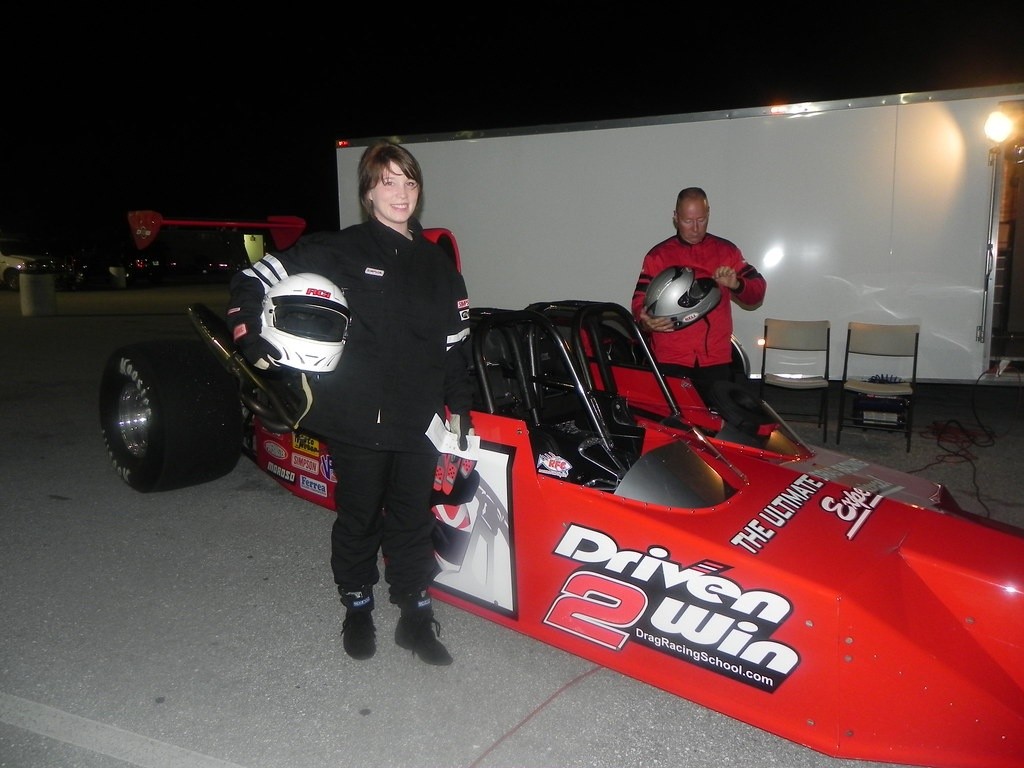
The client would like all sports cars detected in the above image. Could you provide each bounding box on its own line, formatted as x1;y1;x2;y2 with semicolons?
95;291;1024;768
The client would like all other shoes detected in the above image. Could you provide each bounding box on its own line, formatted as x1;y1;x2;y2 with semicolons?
395;606;453;666
341;610;377;660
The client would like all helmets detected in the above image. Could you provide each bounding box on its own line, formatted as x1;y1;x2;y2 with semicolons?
644;266;721;330
259;273;350;377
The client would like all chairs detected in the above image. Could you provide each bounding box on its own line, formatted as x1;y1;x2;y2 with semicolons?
836;321;922;455
759;317;831;444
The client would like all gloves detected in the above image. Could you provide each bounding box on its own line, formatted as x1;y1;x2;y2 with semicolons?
449;411;475;463
226;307;284;372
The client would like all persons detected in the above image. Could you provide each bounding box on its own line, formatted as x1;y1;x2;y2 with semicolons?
225;142;474;666
631;187;768;395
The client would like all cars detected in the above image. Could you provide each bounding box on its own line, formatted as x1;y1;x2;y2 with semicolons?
67;241;170;289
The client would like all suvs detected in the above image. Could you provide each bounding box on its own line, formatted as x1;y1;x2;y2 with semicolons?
1;239;76;294
